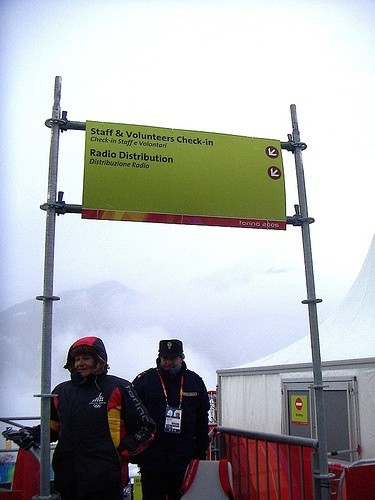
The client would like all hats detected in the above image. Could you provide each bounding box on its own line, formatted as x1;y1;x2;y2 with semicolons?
159;340;183;359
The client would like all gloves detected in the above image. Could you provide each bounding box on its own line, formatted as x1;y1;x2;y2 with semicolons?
6;427;40;451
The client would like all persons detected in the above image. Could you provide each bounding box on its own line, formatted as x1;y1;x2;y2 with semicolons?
12;336;150;500
130;338;212;500
